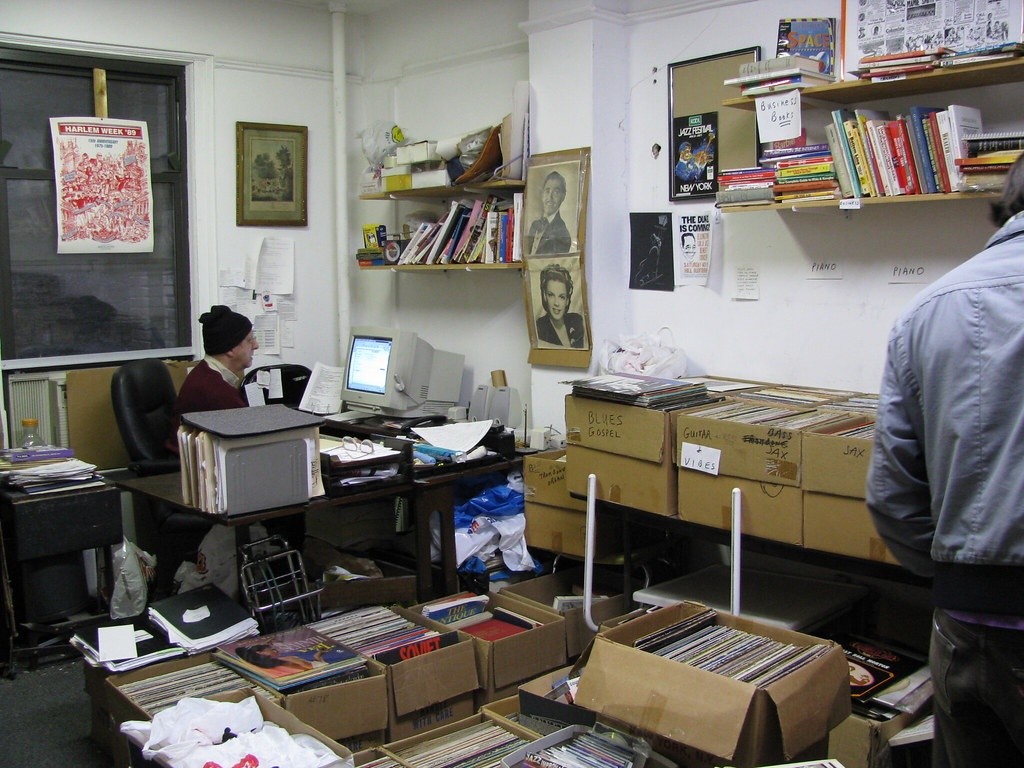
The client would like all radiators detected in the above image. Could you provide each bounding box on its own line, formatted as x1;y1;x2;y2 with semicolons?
6;369;61;449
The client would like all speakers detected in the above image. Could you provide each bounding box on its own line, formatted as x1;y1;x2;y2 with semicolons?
468;384;497;423
489;387;522;428
384;239;411;264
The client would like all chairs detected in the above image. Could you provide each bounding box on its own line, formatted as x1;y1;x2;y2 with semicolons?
551;531;679;608
110;358;215;597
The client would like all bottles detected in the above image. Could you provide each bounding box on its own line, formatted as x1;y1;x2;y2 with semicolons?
15;418;47;448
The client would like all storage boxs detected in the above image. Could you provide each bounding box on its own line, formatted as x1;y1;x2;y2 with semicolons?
319;556;417;611
361;222;387;249
412;169;452;190
381;165;412;192
395;139;441;164
82;374;922;768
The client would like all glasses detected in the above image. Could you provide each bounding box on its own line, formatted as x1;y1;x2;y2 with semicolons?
323;436;373;459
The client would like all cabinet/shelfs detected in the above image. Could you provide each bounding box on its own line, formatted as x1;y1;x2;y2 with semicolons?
358;178;524;273
721;57;1024;213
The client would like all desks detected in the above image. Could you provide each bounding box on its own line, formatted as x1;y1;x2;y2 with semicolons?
115;450;525;605
291;406;456;437
568;472;935;634
0;475;123;672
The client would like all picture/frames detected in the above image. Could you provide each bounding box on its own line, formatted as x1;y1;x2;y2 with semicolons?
667;46;764;202
235;121;308;227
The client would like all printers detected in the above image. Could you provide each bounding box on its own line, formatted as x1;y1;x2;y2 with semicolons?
320;433;413;499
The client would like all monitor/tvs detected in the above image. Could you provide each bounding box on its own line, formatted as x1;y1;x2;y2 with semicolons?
340;326;465;418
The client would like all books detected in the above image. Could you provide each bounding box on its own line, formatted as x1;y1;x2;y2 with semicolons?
557;372;880;439
356;193;524;266
858;42;1024;80
116;569;934;768
723;17;837;97
715;104;1024;209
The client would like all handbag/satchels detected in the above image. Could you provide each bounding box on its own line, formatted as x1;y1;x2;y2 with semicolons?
96;535;147;619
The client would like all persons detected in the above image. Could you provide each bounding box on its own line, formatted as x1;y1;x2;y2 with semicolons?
865;150;1024;768
169;304;326;583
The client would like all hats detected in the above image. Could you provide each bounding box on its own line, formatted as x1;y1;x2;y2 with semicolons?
198;305;252;355
